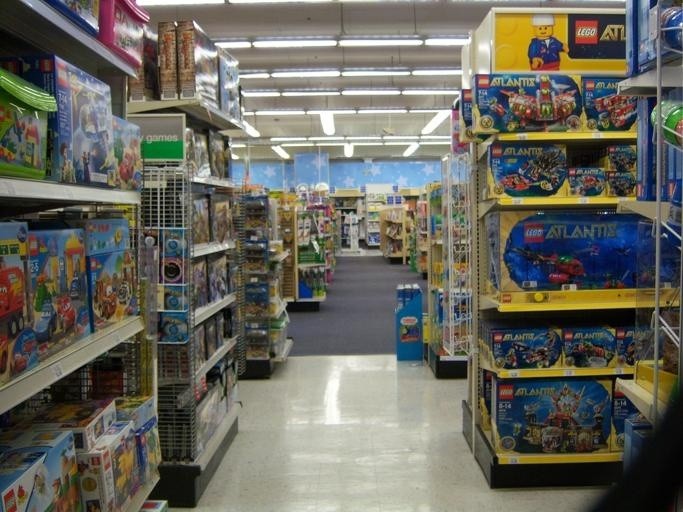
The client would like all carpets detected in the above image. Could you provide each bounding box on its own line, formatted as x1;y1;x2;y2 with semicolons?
280;256;428;356
